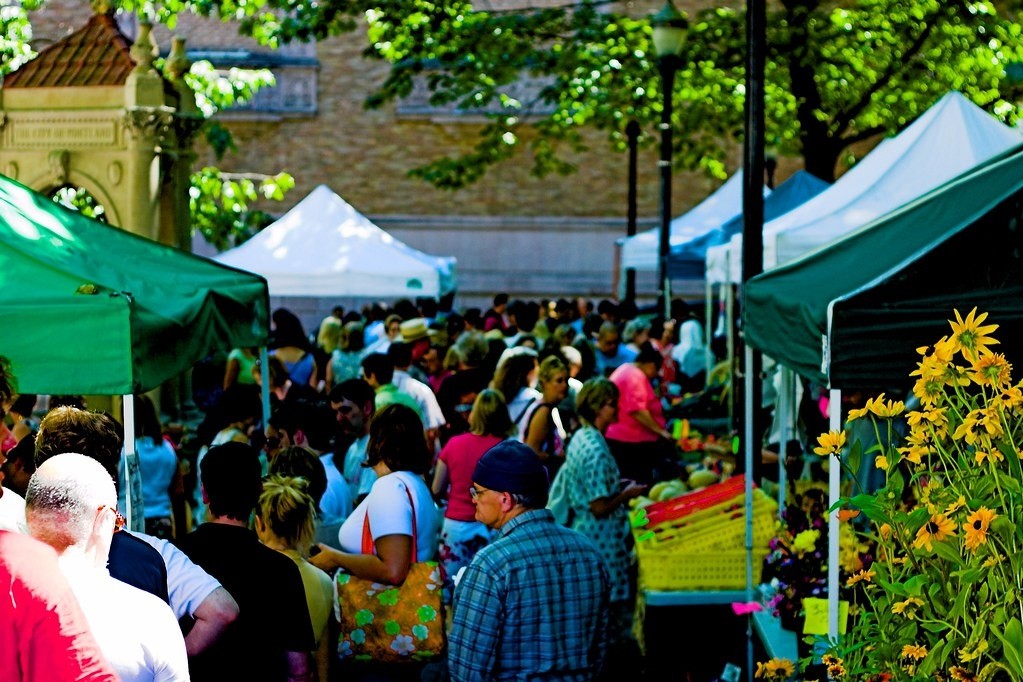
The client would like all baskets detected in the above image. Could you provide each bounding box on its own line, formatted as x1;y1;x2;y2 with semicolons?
628;475;778;590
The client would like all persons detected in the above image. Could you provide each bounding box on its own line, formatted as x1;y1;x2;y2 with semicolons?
0;294;905;682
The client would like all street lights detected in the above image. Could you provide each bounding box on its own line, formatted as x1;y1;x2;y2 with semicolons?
646;0;693;335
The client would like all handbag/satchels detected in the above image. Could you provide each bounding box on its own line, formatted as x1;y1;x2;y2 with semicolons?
336;475;443;661
545;461;570;525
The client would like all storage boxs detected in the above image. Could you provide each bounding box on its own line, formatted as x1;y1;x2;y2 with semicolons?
634;473;776;592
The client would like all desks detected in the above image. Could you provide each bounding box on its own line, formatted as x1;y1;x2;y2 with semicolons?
643;590;801;671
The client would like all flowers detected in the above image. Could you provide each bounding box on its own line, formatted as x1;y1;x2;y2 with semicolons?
750;304;1023;681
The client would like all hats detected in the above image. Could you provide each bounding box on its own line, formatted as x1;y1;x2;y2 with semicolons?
472;439;550;494
400;318;428;339
210;426;252;446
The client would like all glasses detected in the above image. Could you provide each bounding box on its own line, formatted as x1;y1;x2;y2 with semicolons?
98;505;125;532
606;399;618;407
469;486;487;501
257;432;279;449
554;376;569;383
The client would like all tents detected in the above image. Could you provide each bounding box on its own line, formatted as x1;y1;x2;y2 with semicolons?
612;91;1023;682
1;173;273;531
204;183;459;341
